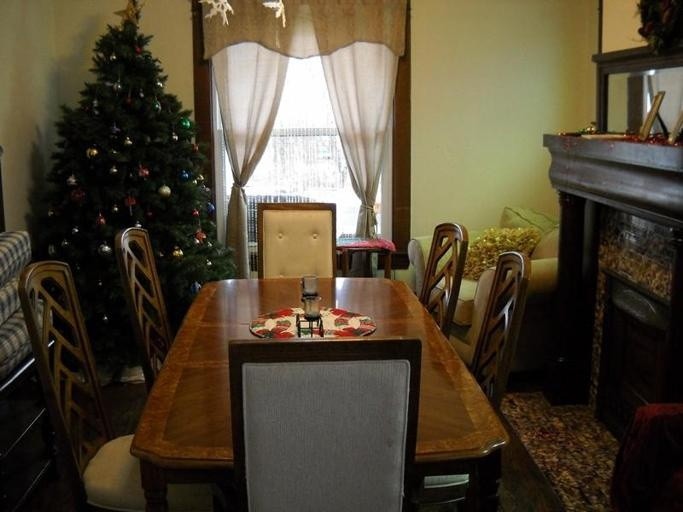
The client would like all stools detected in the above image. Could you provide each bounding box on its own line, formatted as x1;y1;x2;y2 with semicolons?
334;234;397;280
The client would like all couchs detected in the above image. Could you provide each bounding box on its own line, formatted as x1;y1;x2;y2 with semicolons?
407;205;564;394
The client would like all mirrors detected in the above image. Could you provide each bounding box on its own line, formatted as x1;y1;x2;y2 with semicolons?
598;59;682;139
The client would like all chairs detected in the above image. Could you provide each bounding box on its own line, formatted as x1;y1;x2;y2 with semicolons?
422;247;535;512
608;399;683;512
112;223;174;395
17;258;218;511
416;221;477;339
253;200;339;285
224;328;429;512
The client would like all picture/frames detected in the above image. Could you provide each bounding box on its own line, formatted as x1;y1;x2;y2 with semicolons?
637;90;666;142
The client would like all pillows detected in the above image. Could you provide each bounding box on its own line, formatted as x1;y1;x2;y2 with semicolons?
449;226;541;283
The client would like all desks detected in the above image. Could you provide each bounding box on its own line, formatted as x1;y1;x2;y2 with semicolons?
126;273;517;512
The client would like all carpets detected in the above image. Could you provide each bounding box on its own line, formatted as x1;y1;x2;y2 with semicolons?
499;386;621;512
